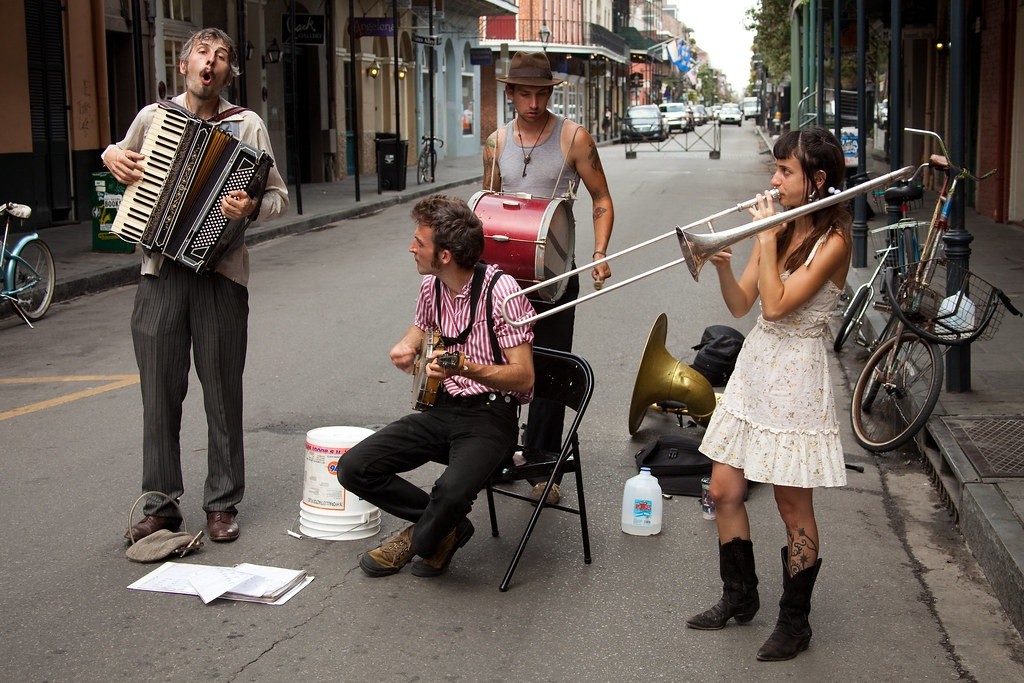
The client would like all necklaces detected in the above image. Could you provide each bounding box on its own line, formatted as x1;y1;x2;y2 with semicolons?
511;112;551;175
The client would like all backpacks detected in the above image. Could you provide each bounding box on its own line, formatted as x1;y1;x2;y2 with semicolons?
633;435;756;498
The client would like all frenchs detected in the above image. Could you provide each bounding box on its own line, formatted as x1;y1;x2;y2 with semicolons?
628;312;716;435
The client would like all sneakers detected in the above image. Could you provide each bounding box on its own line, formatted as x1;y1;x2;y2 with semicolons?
411;517;474;577
359;522;415;577
531;481;562;506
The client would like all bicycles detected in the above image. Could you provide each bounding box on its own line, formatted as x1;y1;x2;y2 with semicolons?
849;127;1024;453
845;160;950;323
416;135;444;185
0;202;56;330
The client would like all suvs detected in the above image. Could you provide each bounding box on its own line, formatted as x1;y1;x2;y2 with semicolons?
718;103;744;128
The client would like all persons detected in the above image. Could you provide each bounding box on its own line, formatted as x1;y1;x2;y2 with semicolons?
483;52;615;507
686;127;854;661
336;193;538;578
100;24;290;540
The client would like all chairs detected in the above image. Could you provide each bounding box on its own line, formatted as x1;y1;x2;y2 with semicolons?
484;343;601;594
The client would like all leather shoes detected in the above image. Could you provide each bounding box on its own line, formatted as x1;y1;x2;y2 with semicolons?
124;515;183;539
206;510;240;540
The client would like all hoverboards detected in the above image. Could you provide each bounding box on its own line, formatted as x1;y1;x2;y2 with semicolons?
832;237;944;353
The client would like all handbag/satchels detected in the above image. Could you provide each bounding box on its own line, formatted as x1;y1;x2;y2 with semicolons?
693;326;745;384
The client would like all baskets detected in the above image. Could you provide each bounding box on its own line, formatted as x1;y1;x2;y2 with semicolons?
869;223;927;267
897;254;1008;344
871;184;924;211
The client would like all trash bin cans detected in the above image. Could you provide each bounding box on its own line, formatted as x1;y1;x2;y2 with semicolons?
373;138;409;191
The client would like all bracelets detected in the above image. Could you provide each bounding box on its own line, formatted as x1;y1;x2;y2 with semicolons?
592;252;607;257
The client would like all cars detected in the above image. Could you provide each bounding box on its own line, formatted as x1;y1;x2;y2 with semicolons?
619;103;669;142
659;103;694;134
690;104;721;126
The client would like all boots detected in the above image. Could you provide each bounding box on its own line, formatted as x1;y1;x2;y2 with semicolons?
686;539;760;630
758;545;823;661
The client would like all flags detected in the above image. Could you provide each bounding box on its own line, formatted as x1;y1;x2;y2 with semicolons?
663;40;693;73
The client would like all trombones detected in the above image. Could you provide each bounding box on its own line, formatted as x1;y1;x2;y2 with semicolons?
503;162;913;328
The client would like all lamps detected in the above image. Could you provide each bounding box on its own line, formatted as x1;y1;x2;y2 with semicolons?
261;37;284;70
398;62;408;80
366;56;382;80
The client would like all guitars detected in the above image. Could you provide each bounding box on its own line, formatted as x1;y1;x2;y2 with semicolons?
410;330;467;411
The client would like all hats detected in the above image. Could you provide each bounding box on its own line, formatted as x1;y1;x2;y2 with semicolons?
495;50;564;87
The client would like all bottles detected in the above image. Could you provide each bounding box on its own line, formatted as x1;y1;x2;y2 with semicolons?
621;467;662;536
935;290;975;340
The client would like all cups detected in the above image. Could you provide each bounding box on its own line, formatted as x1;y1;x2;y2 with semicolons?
701;477;717;521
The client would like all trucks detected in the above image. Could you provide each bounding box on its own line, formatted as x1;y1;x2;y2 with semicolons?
743;96;762;121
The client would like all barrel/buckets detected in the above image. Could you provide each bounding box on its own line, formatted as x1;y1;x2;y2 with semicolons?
286;425;382;541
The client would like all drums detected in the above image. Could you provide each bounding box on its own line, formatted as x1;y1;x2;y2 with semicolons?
466;190;575;304
